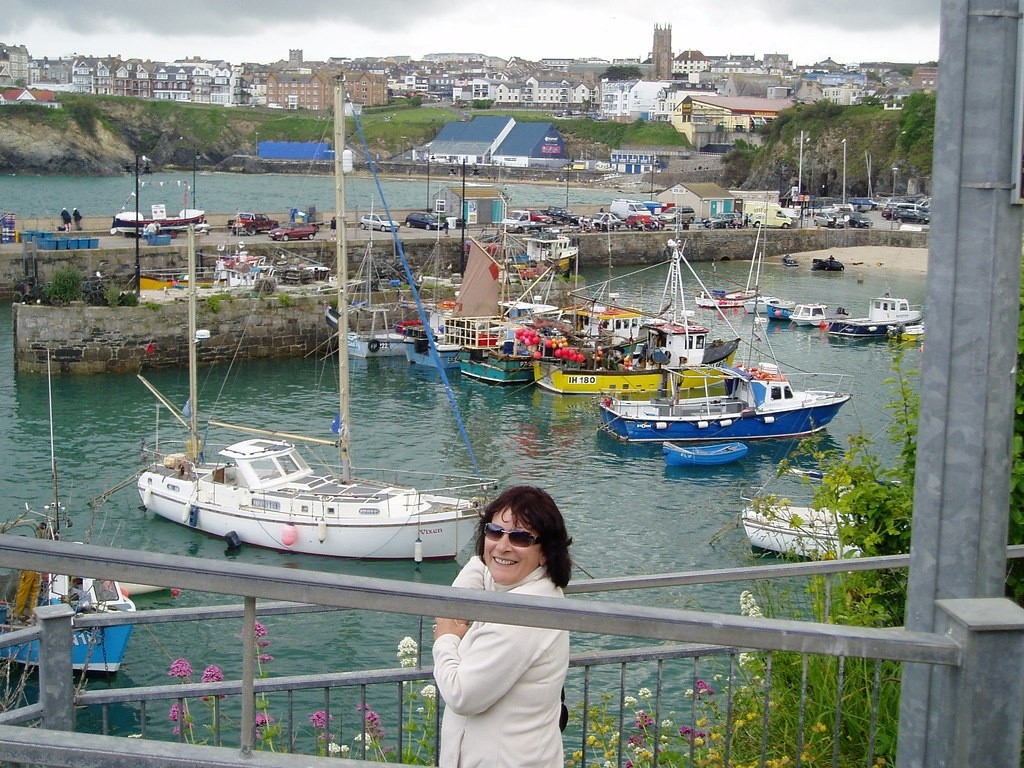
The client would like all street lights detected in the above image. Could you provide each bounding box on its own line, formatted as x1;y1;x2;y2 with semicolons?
890;167;898;229
840;138;848;203
798;131;811;194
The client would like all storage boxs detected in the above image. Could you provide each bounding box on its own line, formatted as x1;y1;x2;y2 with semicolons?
18;231;99;251
1;214;16;244
394;323;434;338
390;279;401;287
758;362;778;375
591;307;605;312
436;335;445;345
520;269;535;280
644;319;665;326
452;273;463;284
150;235;172;246
479;334;497;346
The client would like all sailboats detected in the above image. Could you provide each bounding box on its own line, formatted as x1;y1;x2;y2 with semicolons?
130;88;490;561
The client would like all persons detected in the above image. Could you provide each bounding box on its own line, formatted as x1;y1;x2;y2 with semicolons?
13;569;42;619
331;216;336;240
579;215;594;229
432;486;573;768
61;208;71;232
73;208;82;231
143;220;160;246
444;220;449;236
600;206;604;213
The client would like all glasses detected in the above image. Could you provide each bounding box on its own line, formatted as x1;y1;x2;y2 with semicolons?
484;521;543;547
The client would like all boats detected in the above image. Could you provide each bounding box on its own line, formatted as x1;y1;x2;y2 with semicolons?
788;302;852;328
783;254;799;267
740;503;861;560
112;251;375;305
413;234;588;292
0;343;135;676
110;208;205;235
694;288;796;320
347;281;742;395
886;322;925;341
662;440;749;467
824;289;924;339
596;362;857;443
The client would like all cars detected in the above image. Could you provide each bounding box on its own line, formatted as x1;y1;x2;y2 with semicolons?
268;221;316;241
525;199;744;231
813;201;930;229
227;212;279;236
405;212;445;231
359;213;401;232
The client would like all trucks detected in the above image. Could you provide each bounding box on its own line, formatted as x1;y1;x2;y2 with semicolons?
743;201;794;230
500;210;548;233
